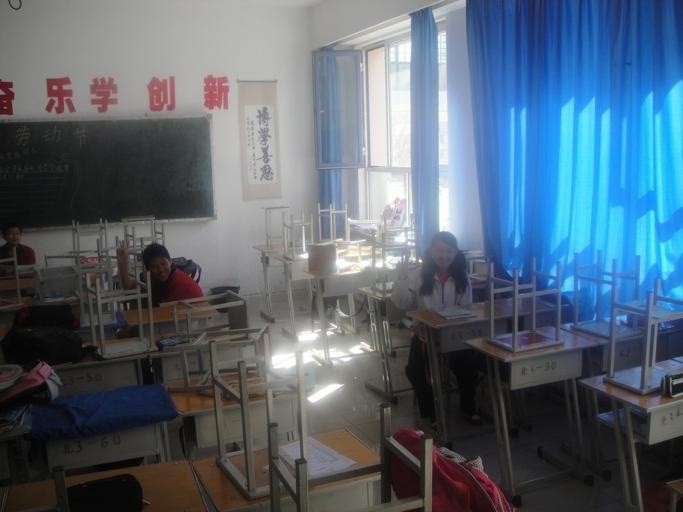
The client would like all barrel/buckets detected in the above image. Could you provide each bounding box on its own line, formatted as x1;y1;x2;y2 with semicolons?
307;242;338;275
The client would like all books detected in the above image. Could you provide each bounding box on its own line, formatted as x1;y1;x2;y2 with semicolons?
434;305;477;319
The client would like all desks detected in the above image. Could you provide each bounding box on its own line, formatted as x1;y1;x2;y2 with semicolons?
267;203;682;394
251;244;682;511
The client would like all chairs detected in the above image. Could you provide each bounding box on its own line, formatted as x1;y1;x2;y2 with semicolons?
0;217;435;512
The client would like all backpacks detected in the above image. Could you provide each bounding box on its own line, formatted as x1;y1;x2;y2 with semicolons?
0;304;106;406
379;197;407;237
169;257;202;283
388;427;523;512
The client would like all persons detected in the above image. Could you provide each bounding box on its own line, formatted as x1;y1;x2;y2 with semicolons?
392;231;480;429
118;240;203;305
0;224;36;265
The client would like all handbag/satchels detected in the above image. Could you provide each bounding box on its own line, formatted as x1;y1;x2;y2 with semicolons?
67;473;143;511
